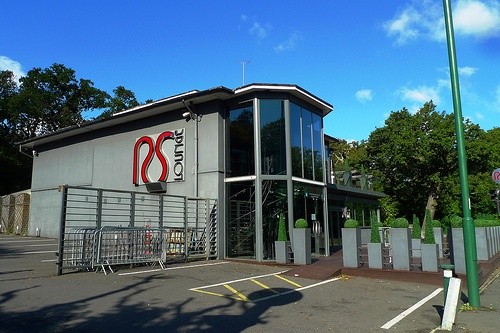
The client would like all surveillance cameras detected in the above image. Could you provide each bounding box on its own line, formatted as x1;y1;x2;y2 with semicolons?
32;150;38;156
182;112;191;118
